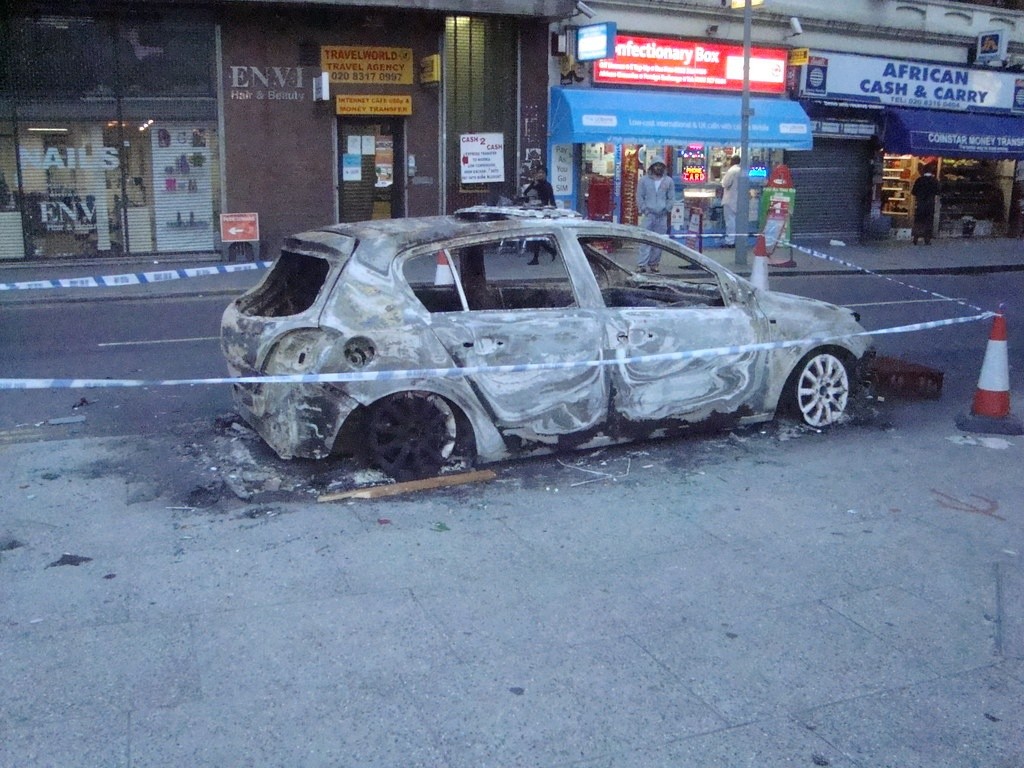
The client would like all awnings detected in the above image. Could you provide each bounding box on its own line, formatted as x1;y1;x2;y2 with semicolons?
550;85;814;151
881;105;1024;161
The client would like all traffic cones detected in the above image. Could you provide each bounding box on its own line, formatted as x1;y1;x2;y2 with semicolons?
431;248;455;286
952;304;1024;438
749;236;771;291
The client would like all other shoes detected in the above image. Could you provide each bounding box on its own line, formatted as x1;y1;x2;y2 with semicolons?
527;259;539;265
720;243;735;248
635;266;646;273
552;249;557;261
650;266;659;272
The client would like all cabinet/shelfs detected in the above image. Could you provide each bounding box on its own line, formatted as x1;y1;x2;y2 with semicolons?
881;157;911;215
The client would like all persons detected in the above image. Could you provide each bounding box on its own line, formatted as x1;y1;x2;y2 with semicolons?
719;155;750;248
524;165;557;265
911;164;945;246
635;156;675;273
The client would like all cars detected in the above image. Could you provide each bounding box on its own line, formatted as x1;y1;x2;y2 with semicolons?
214;203;880;480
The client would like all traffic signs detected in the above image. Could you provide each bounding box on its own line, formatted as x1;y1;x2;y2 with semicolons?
218;213;261;243
